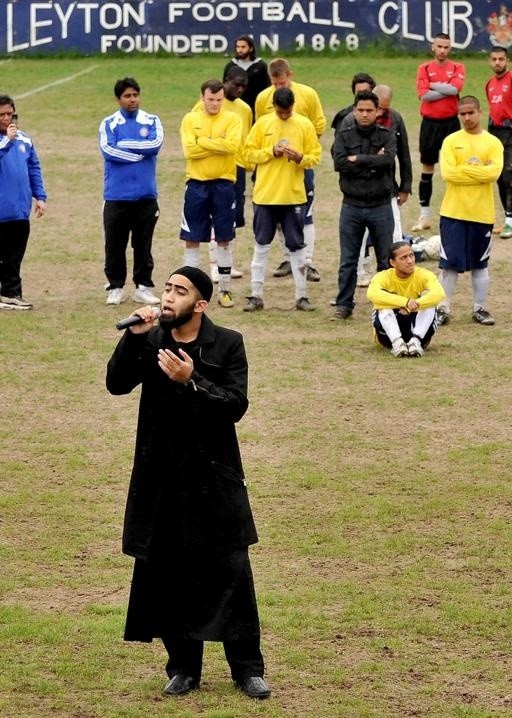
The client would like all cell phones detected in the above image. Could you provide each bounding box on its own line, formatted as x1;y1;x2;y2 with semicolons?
11;113;18;126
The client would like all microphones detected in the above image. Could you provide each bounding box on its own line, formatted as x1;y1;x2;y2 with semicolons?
116;307;161;330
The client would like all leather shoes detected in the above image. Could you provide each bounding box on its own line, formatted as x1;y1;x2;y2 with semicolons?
164;674;195;694
242;677;271;697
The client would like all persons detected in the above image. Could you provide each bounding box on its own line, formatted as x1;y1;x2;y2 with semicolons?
364;242;449;358
332;72;377;272
254;58;323;282
331;91;399;319
181;77;237;308
104;265;270;699
190;67;253;283
485;46;512;239
223;35;272;182
1;92;49;310
436;94;503;328
98;76;162;307
332;83;416;308
411;34;465;231
243;87;321;313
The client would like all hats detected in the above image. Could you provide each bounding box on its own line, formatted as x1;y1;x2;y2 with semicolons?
170;266;213;301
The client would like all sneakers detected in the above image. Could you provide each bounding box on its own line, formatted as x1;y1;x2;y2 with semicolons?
331;310;351;321
412;220;430;231
106;288;126;305
212;267;242;283
135;284;160;304
391;336;423;357
500;225;512;238
274;262;320;281
244;297;263;311
218;291;234;308
0;296;32;310
296;297;314;310
473;307;495;325
